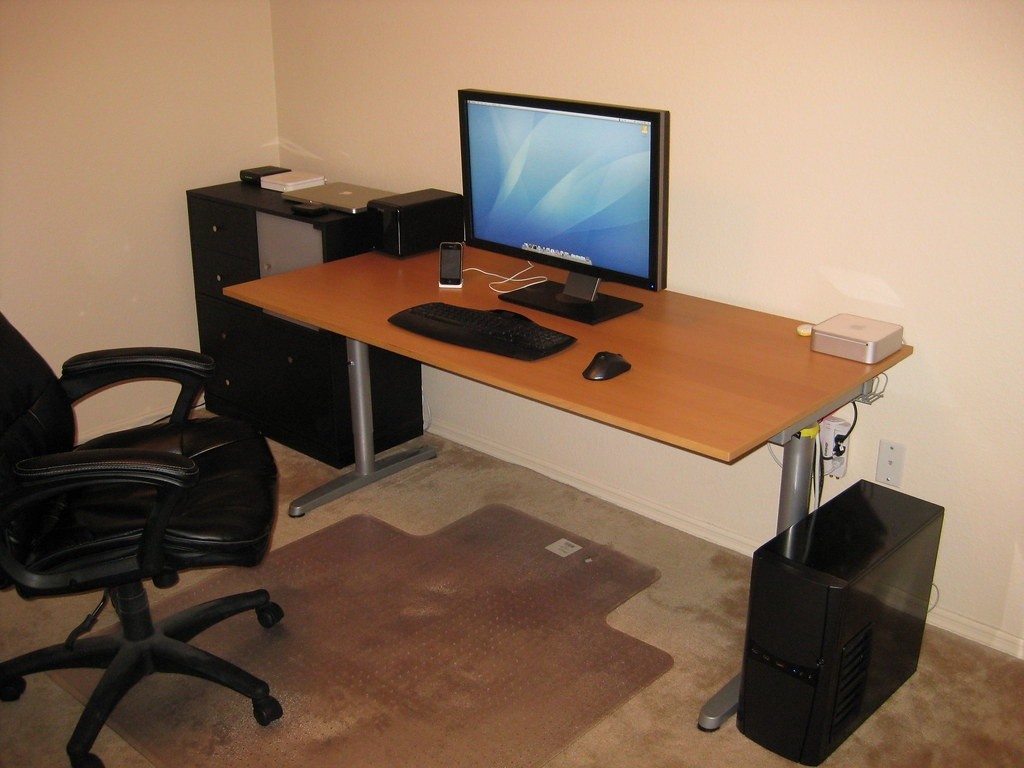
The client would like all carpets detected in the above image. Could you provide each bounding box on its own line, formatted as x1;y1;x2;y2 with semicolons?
34;500;675;768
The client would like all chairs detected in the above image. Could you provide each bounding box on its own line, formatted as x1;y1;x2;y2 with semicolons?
0;314;288;768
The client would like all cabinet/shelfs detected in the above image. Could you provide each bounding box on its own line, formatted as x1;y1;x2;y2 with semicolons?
187;182;425;470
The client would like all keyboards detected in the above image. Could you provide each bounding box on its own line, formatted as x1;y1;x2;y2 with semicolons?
387;302;577;362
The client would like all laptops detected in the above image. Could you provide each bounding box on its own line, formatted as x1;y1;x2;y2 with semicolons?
281;182;400;214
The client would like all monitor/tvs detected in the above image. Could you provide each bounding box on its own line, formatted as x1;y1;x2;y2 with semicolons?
459;89;671;325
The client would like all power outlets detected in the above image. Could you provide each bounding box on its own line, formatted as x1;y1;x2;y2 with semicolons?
819;416;851;478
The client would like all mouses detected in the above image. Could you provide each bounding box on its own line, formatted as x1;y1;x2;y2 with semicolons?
582;351;632;380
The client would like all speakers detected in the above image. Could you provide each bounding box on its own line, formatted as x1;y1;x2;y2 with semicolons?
366;188;465;257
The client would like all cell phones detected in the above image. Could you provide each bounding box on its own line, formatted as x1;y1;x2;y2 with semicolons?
440;242;464;285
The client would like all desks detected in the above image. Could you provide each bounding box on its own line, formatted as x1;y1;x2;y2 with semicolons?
223;239;916;731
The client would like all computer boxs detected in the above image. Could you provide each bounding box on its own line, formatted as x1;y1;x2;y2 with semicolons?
734;478;946;767
